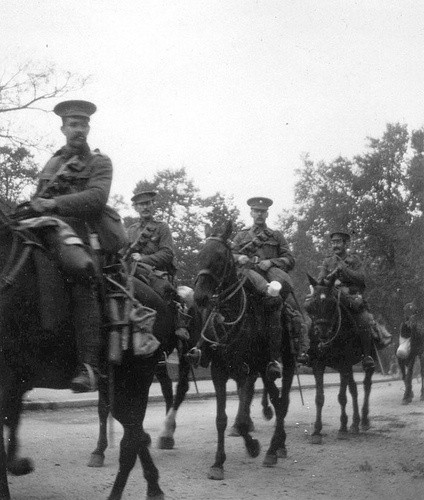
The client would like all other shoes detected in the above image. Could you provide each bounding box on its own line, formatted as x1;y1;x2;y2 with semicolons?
297;352;310;364
268;361;284;378
361;356;375;370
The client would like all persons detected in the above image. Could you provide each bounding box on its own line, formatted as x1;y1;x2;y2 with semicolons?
123;184;189;340
30;100;112;389
232;197;296;380
299;233;375;369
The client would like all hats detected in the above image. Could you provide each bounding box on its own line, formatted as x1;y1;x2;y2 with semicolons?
53;100;97;118
131;191;157;205
330;232;350;240
247;197;273;210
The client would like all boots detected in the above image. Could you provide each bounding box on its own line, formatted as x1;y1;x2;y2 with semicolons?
71;283;105;392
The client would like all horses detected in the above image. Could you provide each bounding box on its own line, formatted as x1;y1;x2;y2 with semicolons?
390;304;424;407
302;267;383;445
192;216;306;481
0;189;195;500
